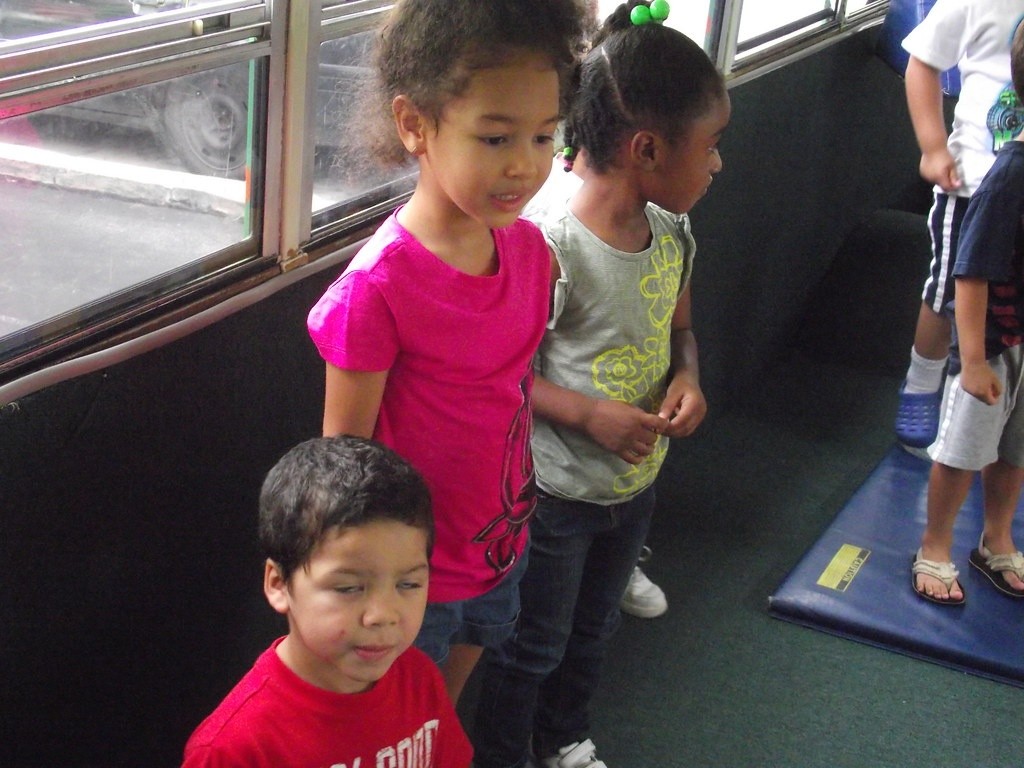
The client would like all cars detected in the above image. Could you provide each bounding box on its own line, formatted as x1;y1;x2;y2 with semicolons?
0;0;377;179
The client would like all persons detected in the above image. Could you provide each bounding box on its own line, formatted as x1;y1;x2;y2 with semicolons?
894;0;1024;448
472;0;731;768
307;0;584;711
911;20;1024;605
182;432;475;768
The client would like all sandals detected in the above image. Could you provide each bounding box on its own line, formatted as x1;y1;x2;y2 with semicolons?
911;547;966;607
969;532;1024;598
894;358;948;450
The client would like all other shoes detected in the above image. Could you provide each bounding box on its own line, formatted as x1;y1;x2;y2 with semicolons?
544;739;607;768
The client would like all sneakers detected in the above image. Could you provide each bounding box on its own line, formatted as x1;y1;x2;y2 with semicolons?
621;566;667;620
